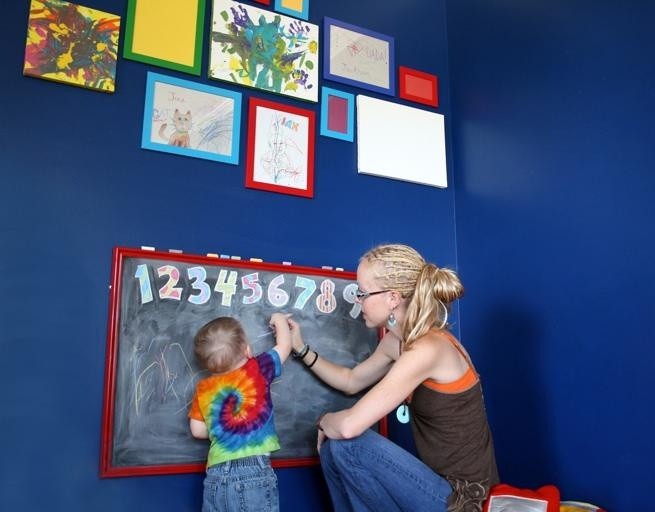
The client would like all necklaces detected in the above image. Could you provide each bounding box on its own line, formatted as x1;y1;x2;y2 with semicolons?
396;339;410;425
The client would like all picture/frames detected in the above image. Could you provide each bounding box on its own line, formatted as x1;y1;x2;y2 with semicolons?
243;94;317;200
273;0;312;21
397;64;441;108
319;85;356;145
124;1;206;75
321;14;399;100
138;70;244;167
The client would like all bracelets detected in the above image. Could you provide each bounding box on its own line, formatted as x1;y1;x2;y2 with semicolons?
292;344;318;368
315;409;332;429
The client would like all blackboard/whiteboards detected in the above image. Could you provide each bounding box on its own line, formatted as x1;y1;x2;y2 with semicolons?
100;247;390;479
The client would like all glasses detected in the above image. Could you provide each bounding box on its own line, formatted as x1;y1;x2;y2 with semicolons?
356;289;390;303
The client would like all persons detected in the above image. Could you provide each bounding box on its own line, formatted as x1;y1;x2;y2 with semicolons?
188;312;293;512
273;244;501;512
